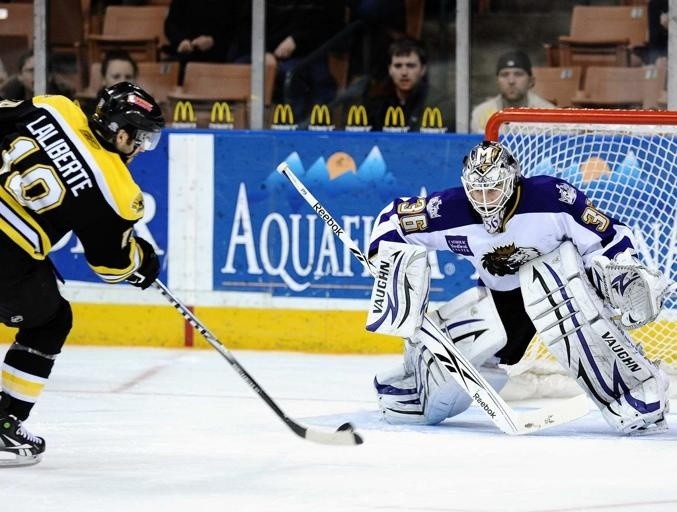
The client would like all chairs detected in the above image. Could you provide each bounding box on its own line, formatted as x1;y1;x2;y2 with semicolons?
0;0;425;129
531;0;668;109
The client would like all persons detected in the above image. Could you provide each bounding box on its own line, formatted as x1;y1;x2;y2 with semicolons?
469;51;561;133
151;1;255;65
4;50;72;101
1;80;168;464
640;1;667;111
355;142;670;433
262;0;353;128
92;49;138;103
357;38;453;133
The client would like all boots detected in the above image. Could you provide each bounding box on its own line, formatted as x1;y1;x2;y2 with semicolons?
1;393;46;456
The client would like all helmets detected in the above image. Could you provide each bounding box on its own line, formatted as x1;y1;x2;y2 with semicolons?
89;80;166;161
459;139;519;235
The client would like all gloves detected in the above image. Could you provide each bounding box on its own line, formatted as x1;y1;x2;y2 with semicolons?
127;235;160;291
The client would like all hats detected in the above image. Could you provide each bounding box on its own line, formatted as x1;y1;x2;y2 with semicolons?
495;50;533;78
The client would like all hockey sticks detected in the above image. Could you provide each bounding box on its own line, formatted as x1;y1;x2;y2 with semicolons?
276;162;592;435
152;278;366;445
